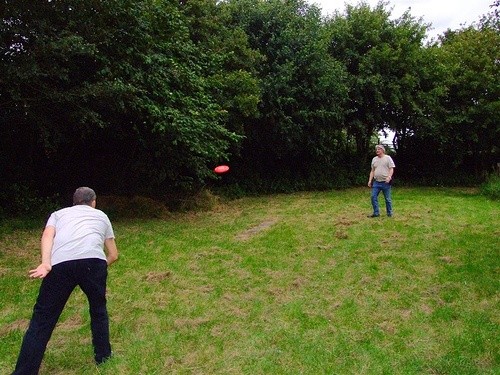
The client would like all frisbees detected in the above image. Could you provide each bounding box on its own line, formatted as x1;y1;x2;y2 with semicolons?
214;165;229;173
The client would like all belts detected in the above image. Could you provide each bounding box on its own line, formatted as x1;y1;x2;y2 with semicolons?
378;181;385;183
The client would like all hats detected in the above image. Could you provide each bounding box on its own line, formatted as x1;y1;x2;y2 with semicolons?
375;144;385;153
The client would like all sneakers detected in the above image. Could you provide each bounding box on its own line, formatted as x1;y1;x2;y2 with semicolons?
367;213;380;218
388;213;393;217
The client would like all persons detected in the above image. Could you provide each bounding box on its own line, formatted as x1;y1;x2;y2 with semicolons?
13;187;119;375
367;144;396;218
